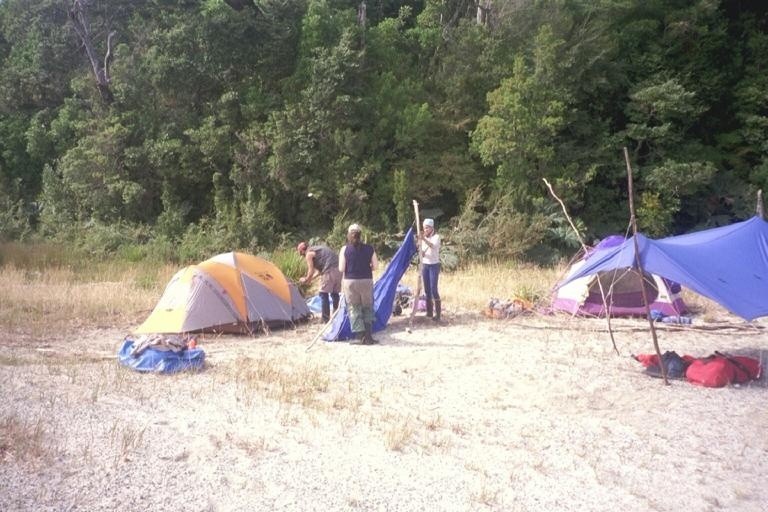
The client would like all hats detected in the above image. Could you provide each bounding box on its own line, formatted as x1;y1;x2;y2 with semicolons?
347;223;362;233
297;241;308;256
423;218;434;227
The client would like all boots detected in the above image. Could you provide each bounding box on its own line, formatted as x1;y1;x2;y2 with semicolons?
356;323;379;345
425;298;441;321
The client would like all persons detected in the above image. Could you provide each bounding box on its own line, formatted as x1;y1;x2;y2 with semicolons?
298;242;343;324
416;218;441;320
339;224;378;344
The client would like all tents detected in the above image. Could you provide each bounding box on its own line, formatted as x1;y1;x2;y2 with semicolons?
132;251;310;334
550;216;768;319
